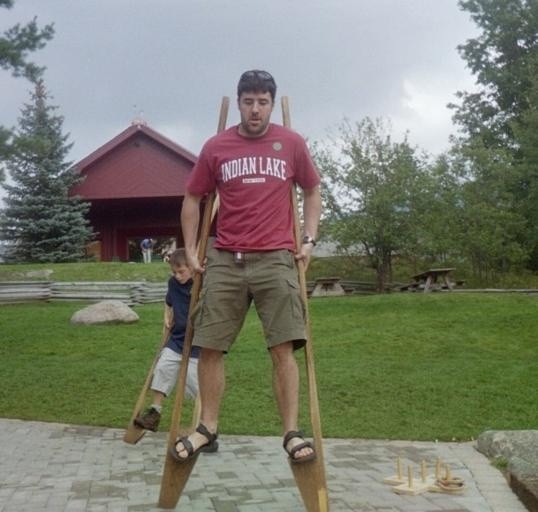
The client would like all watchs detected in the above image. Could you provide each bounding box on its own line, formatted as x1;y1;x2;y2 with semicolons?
303;235;316;246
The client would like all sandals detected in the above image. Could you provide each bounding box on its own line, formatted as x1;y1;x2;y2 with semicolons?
283;431;315;464
171;424;218;462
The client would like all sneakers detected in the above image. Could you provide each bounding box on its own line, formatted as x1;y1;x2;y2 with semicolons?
135;407;160;432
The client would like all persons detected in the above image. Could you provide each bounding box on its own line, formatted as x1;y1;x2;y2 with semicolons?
140;238;153;263
161;248;173;262
134;247;201;433
169;69;324;465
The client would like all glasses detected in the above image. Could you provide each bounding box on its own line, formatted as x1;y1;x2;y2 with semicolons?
239;70;272;82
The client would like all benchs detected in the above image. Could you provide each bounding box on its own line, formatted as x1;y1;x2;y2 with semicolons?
408;267;457;294
